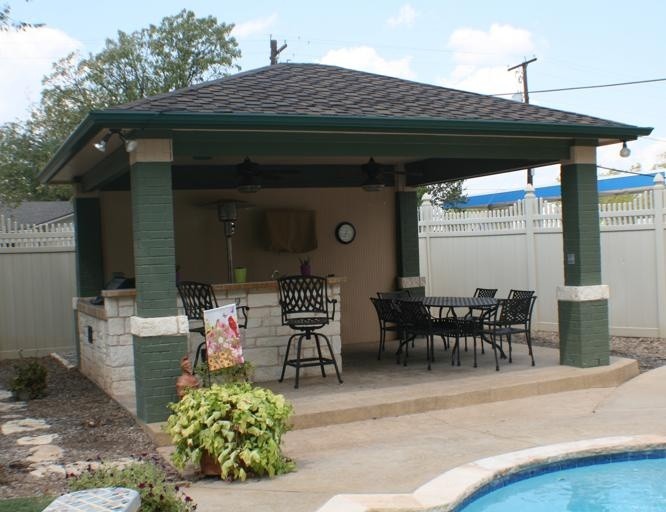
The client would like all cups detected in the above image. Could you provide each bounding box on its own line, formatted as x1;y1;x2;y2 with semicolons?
233;266;246;283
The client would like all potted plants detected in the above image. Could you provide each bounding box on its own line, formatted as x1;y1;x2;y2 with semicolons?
161;380;299;482
6;360;48;401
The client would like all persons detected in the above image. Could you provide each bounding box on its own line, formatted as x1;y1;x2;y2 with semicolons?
174;354;200;396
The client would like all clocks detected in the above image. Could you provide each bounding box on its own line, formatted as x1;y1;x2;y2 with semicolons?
335;222;357;244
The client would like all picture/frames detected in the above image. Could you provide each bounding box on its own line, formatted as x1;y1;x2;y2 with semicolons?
202;302;245;372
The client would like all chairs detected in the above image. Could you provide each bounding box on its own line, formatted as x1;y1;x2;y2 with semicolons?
176;281;251;378
369;289;536;371
276;276;343;388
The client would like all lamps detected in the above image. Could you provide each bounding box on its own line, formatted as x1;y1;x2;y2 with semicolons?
234;156;267;193
621;140;631;157
93;130;112;152
523;168;539;198
360;157;387;191
119;132;138;153
